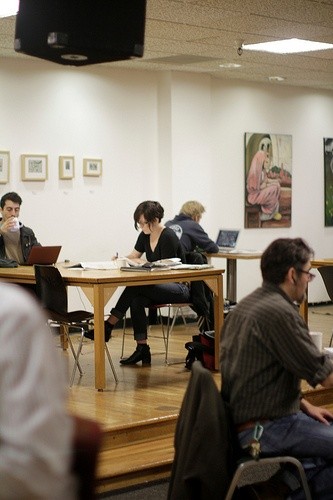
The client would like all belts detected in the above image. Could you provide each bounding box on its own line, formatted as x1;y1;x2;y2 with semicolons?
230;420;271;434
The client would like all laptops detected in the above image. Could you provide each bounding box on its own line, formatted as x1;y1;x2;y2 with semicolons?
20;246;63;266
217;229;240;250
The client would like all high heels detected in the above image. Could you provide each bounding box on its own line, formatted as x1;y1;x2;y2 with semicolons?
119;343;151;365
84;321;114;342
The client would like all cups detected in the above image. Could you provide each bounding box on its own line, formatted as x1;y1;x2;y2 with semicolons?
9;217;24;233
309;331;324;353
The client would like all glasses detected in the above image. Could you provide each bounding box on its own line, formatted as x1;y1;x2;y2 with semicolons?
287;266;315;281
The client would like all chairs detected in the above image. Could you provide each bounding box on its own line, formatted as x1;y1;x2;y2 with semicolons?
32;265;197;383
192;360;312;499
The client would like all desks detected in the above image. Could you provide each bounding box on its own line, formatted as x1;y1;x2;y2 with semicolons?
0;260;225;392
205;252;333;328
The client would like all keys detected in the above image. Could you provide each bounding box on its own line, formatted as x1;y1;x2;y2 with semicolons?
251;442;260;456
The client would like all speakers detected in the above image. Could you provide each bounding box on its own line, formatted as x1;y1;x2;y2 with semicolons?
14;0;147;67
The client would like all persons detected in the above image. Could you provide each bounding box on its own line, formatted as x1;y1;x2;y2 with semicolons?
218;238;332;500
84;201;191;366
0;192;41;265
164;201;237;330
0;282;71;500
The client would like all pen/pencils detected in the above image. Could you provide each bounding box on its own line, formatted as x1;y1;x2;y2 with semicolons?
116;252;118;258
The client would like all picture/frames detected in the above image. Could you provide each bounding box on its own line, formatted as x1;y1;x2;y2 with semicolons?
59;155;74;180
0;150;10;184
22;154;48;182
83;158;103;177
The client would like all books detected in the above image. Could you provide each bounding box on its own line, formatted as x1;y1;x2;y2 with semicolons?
120;263;170;271
